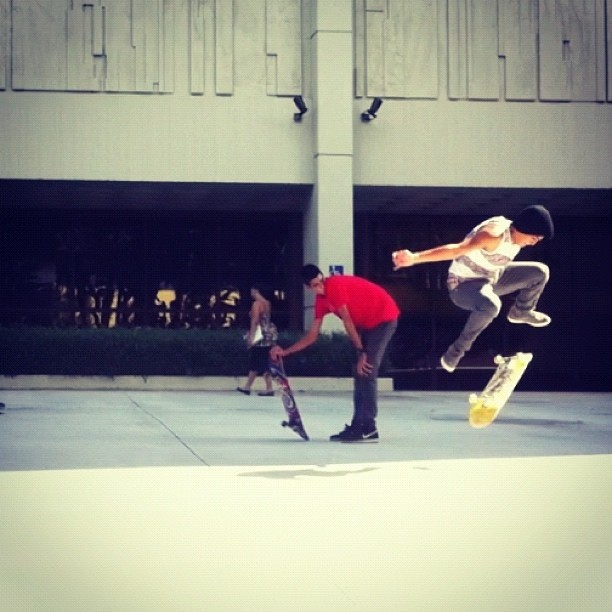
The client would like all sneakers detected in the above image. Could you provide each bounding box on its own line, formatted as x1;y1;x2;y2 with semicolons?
507;308;551;327
440;347;464;373
330;427;379;442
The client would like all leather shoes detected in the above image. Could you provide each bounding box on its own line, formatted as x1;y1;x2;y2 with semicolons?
237;387;250;395
257;391;275;396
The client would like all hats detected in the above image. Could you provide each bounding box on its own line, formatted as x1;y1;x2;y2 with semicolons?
514;205;554;239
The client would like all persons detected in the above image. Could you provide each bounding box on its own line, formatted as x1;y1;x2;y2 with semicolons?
391;205;554;373
236;282;274;396
269;263;400;443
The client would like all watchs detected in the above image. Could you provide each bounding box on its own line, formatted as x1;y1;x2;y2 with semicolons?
355;348;366;354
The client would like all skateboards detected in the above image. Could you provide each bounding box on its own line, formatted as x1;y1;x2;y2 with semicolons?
468;350;533;432
268;353;310;441
